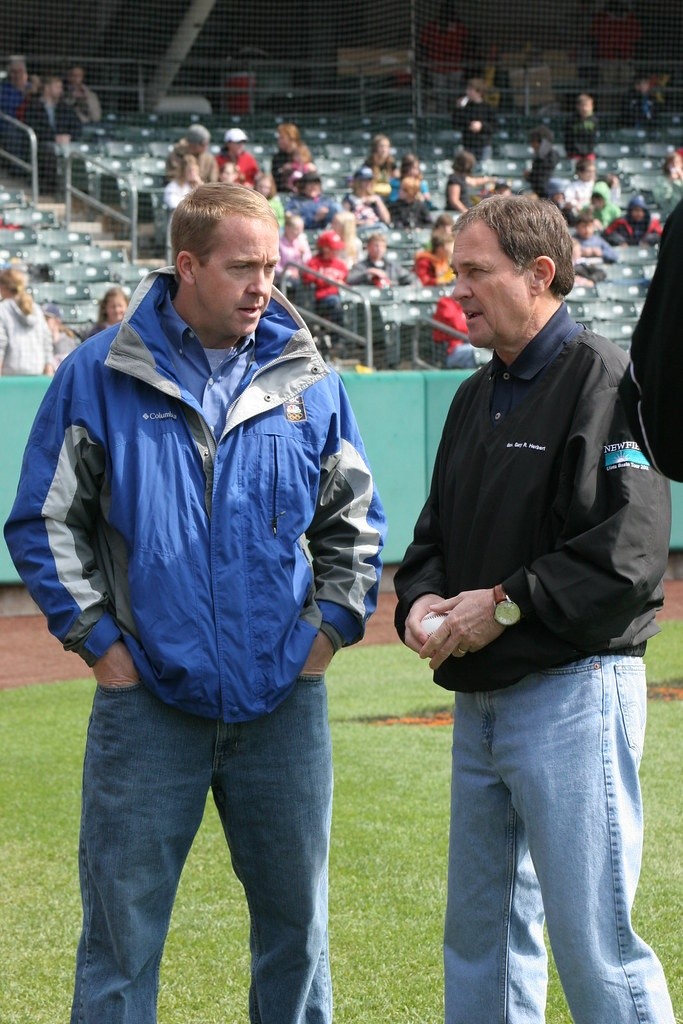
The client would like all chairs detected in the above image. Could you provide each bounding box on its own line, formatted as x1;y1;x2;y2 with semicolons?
0;110;683;375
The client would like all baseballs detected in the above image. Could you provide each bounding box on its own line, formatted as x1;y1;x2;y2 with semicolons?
420;608;451;642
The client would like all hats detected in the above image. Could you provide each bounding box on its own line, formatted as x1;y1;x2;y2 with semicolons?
315;230;346;251
187;124;211;144
300;173;321;183
223;128;247;144
353;165;376;181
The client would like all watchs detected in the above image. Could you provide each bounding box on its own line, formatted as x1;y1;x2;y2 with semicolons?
489;582;523;626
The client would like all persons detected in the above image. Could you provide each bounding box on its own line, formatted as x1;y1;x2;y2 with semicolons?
0;58;104;194
388;185;668;1024
1;264;55;380
85;287;130;339
9;176;388;1024
41;301;80;371
159;65;683;372
